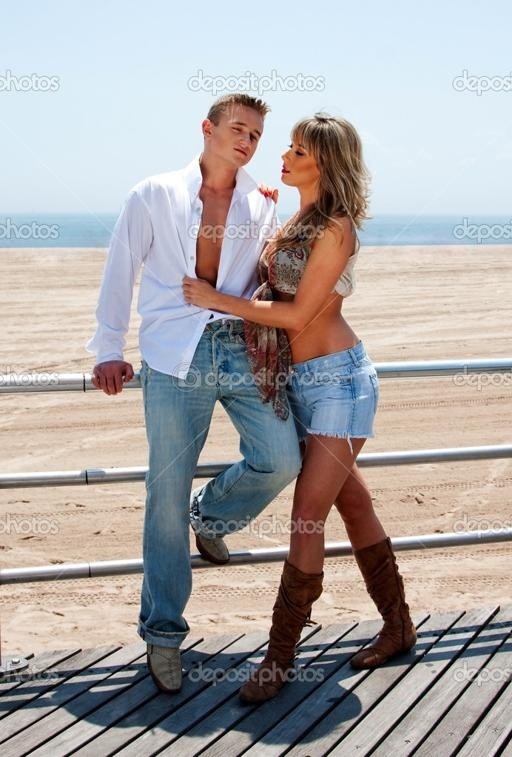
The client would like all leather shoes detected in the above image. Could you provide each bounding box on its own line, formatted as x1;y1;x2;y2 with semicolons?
192;528;234;566
146;646;182;696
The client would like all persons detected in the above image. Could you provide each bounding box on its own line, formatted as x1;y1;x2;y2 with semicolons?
180;110;419;704
84;92;287;695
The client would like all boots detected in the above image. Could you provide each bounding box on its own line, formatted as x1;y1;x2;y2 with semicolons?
352;538;418;671
237;558;323;710
